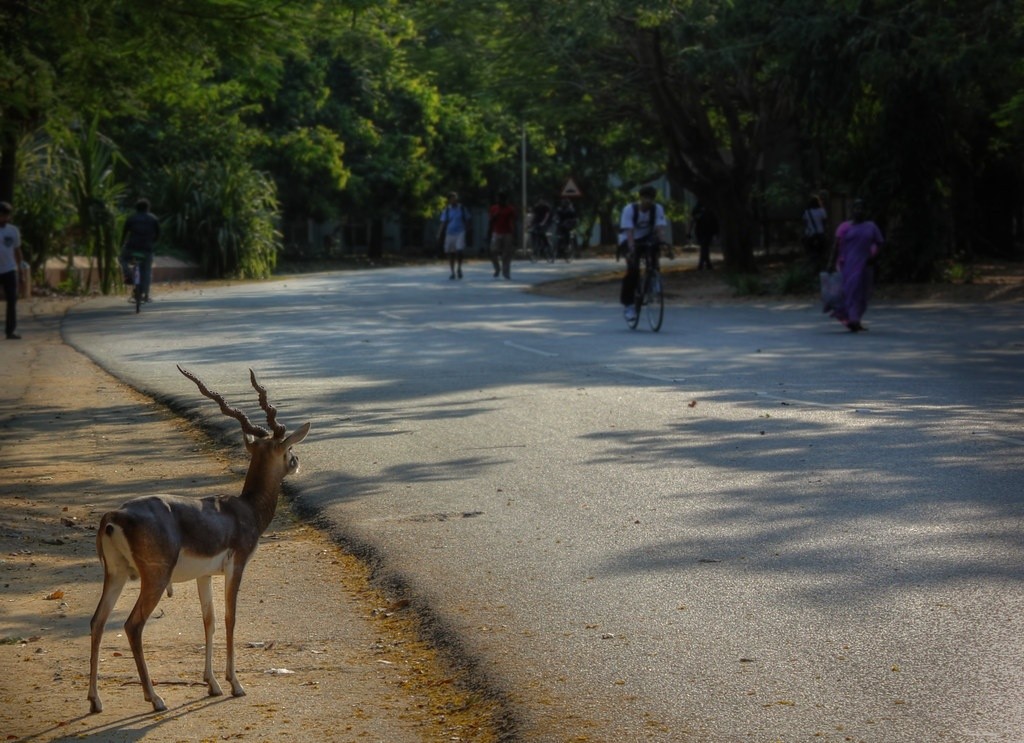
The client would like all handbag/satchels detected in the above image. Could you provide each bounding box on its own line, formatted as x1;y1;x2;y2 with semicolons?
819;271;846;313
812;234;827;248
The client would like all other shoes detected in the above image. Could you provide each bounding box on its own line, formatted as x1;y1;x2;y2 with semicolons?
502;273;510;278
494;268;500;277
624;303;637;320
6;333;22;339
450;274;455;279
458;270;463;278
850;322;867;332
124;275;135;284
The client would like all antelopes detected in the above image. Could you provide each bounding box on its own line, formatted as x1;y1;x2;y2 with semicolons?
85;363;312;713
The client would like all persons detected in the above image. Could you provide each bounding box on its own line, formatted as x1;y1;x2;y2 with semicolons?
435;191;473;280
691;202;720;272
822;199;888;331
803;196;828;258
617;186;670;321
528;196;579;262
117;200;161;301
0;202;23;339
485;193;519;279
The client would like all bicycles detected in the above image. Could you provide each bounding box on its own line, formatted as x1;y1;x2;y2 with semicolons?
619;237;671;333
526;226;577;264
122;251;149;312
